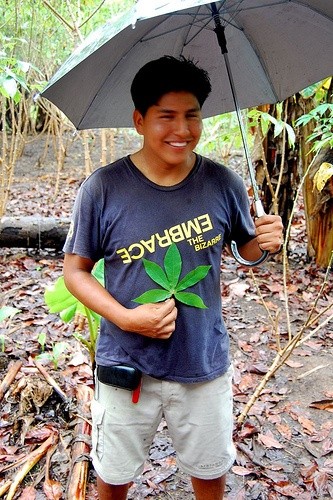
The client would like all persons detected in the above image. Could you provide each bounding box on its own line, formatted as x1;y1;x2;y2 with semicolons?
61;56;285;500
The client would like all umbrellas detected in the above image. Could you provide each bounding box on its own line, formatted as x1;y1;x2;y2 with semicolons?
40;0;333;268
